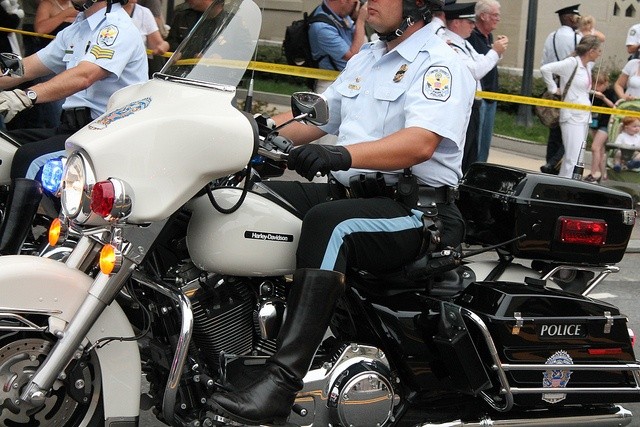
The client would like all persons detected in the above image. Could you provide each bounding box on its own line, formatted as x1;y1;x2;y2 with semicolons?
613;59;640;101
467;1;500;162
574;16;604;45
152;0;251;80
612;116;640;173
626;24;640;59
121;0;170;56
0;0;25;53
284;0;369;95
583;65;617;183
34;0;80;48
540;33;602;180
0;0;149;256
539;4;583;175
444;1;509;175
206;0;477;425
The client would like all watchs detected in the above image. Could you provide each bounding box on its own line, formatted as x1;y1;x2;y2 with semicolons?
22;88;37;104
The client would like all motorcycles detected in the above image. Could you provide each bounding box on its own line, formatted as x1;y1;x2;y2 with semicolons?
1;29;95;252
1;0;638;424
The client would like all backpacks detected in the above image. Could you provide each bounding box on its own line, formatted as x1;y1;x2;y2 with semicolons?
282;6;343;84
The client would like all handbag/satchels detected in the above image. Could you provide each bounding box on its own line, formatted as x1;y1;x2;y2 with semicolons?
534;91;560;128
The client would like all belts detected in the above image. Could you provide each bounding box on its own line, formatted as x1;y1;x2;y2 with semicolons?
432;187;459;206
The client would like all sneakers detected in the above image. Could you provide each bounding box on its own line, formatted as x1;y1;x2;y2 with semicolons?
613;165;621;174
627;159;639;169
585;172;603;183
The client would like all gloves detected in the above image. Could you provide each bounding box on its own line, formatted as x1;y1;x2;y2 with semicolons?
287;143;351;182
1;89;33;124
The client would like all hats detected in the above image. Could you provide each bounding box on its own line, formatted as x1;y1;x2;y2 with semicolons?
442;1;477;24
555;3;581;17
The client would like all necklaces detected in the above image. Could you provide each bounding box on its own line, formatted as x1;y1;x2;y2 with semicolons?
56;1;71;10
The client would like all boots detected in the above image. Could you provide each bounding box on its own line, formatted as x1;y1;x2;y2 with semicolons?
1;178;44;254
208;267;346;425
